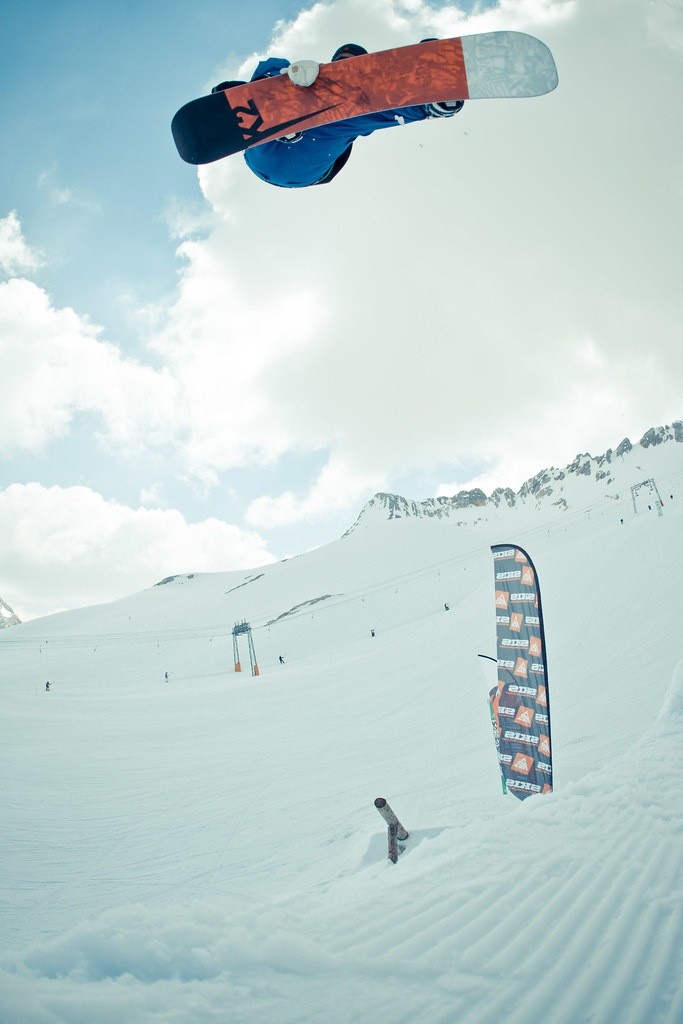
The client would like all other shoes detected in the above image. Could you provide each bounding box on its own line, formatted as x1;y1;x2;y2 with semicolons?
417;39;465;117
211;81;303;144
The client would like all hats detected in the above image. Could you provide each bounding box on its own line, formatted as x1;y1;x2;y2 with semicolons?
333;43;367;61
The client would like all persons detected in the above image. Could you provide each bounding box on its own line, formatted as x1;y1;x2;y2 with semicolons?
244;38;463;189
46;682;51;691
371;630;374;637
279;656;284;663
165;672;169;682
648;505;651;510
670;495;673;499
445;604;449;610
621;519;623;523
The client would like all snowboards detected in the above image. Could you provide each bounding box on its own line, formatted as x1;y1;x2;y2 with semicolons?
171;30;559;165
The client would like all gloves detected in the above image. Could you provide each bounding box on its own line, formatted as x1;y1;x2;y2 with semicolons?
280;60;323;88
